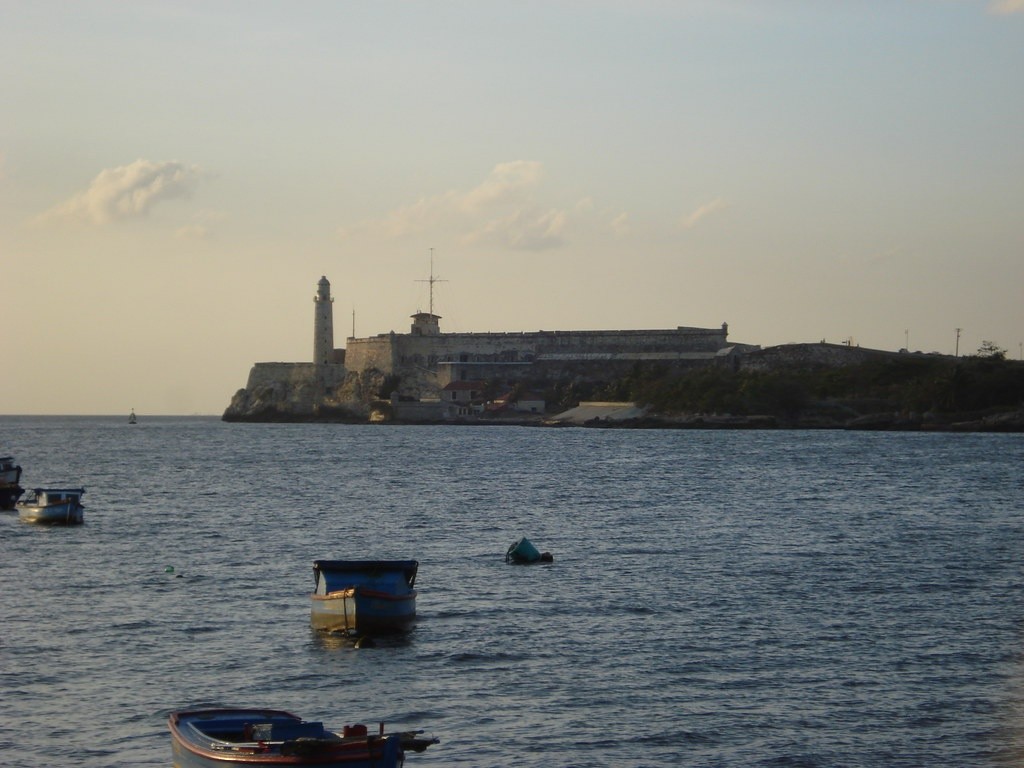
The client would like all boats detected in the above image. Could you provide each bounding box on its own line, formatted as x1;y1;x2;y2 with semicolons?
310;558;421;650
0;455;25;508
165;706;440;768
14;486;87;526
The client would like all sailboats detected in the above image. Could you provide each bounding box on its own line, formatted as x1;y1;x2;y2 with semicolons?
128;408;138;424
